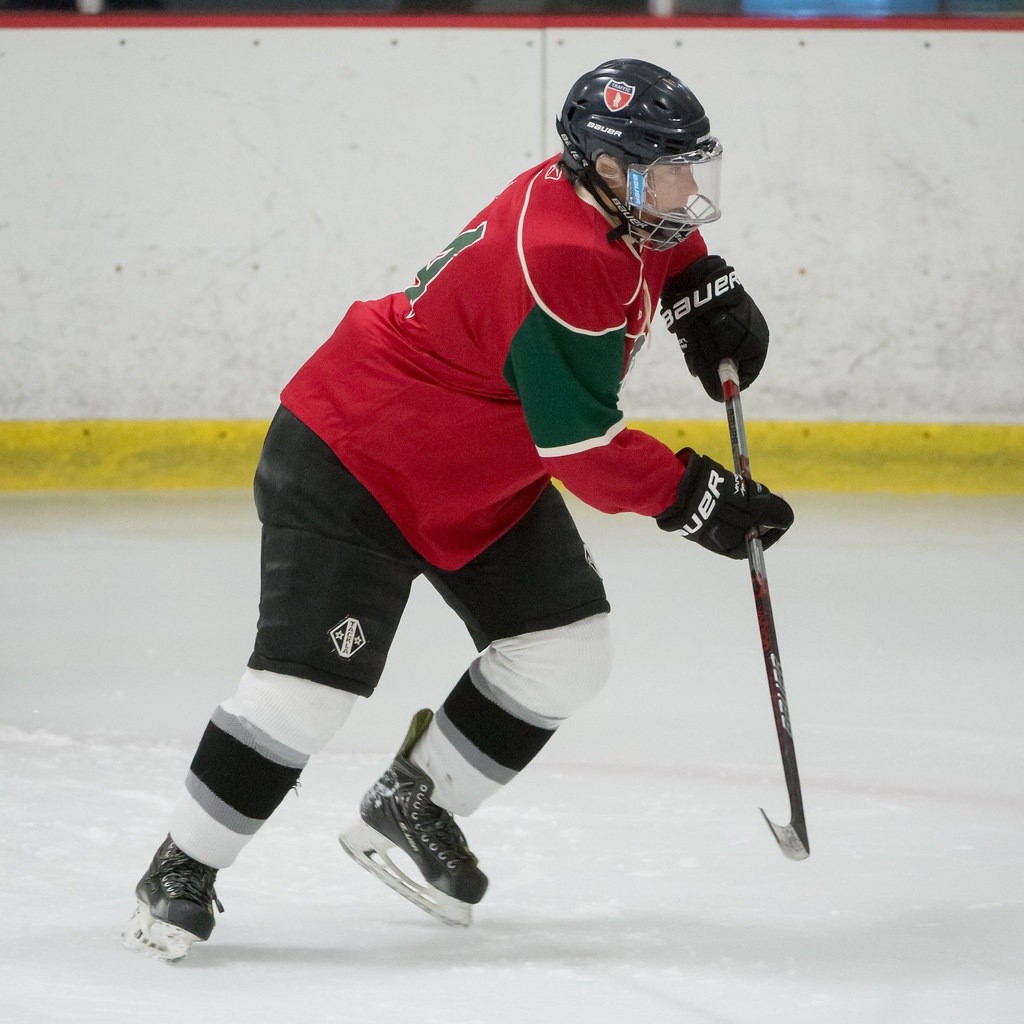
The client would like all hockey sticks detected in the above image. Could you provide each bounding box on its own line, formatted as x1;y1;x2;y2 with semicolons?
718;356;809;863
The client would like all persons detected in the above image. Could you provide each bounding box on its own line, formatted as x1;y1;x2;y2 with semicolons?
119;57;794;964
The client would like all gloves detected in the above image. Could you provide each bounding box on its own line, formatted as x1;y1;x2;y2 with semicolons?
650;446;794;560
660;256;770;402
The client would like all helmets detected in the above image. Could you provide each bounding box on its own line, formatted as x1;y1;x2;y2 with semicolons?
555;57;724;252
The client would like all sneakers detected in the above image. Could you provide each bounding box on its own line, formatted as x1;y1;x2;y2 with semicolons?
112;838;232;963
336;707;488;930
